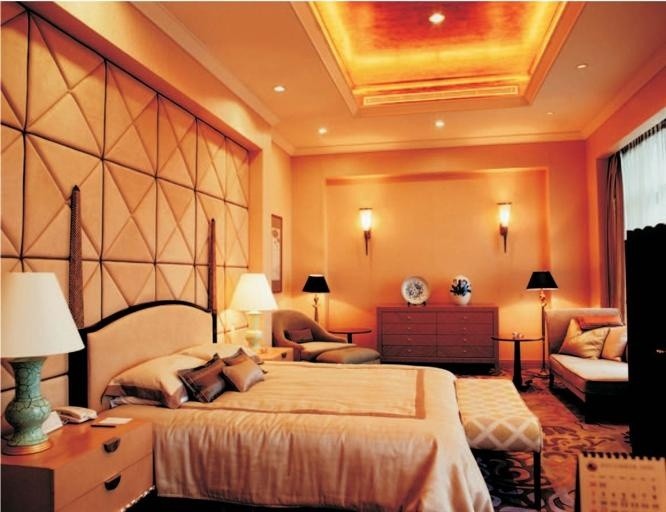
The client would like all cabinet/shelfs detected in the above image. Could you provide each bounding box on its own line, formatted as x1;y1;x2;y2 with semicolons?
377;304;499;367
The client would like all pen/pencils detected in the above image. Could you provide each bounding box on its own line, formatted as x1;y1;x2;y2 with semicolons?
91;425;116;427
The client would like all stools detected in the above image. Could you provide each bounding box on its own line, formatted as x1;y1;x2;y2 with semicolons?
317;347;381;366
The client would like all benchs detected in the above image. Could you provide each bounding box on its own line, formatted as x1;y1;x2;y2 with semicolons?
456;379;543;512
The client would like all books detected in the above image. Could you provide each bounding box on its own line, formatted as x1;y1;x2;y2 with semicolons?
99;416;133;425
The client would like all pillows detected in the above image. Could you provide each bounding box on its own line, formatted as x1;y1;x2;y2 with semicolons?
286;328;314;342
559;317;628;362
106;342;269;406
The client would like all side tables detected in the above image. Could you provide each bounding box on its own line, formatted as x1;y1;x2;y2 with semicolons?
492;332;544;391
329;327;372;343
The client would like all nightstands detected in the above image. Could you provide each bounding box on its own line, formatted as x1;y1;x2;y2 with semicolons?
0;416;156;512
258;346;293;361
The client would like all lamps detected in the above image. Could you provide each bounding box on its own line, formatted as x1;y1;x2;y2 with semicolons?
359;207;374;253
527;271;558;378
301;274;331;322
231;272;278;353
498;202;511;251
0;272;86;455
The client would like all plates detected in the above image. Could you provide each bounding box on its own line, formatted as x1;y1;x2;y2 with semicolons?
401;275;432;305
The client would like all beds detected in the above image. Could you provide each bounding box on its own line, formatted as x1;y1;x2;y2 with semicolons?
70;186;493;512
544;308;628;421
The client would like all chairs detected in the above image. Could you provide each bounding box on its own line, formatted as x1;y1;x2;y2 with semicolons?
272;310;357;360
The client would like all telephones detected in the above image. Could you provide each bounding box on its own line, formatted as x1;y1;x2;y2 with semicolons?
51;406;97;423
